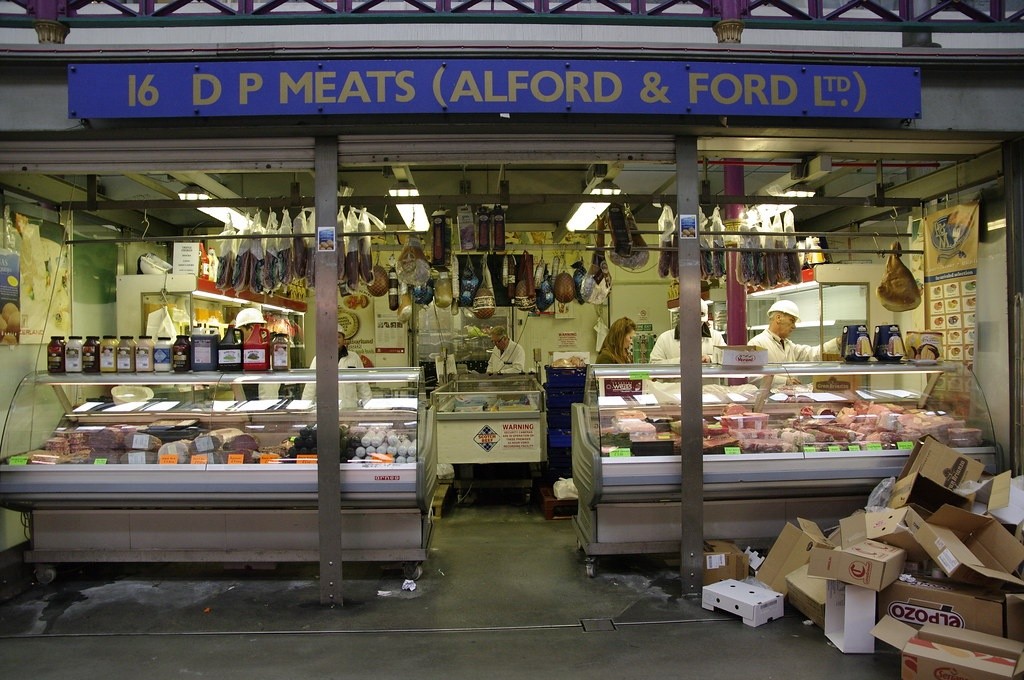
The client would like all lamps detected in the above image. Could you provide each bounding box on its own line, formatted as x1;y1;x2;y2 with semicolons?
566;182;621;235
178;184;259;232
388;183;431;234
747;186;814;228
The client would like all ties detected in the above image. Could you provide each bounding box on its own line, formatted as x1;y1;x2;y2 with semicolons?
501;350;504;355
780;339;784;350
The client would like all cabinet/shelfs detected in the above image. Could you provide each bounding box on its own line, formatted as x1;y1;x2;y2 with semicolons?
747;264;894;346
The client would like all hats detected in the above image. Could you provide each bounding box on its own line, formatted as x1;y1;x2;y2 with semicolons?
337;324;345;335
700;298;708;322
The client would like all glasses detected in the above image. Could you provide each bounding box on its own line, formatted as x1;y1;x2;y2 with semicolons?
491;336;504;345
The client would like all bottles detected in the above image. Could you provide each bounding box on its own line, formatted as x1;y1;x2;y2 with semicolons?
208;247;219;282
46;323;292;373
199;241;210;279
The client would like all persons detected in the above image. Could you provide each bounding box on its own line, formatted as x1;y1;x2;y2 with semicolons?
300;323;373;408
486;325;525;373
747;300;843;363
596;316;636;365
649;299;726;363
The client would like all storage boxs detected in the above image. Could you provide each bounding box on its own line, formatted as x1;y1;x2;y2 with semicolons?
700;433;1024;680
546;361;592;478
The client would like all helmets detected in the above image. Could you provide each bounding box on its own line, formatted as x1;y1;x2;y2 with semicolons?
235;308;267;328
767;300;801;324
